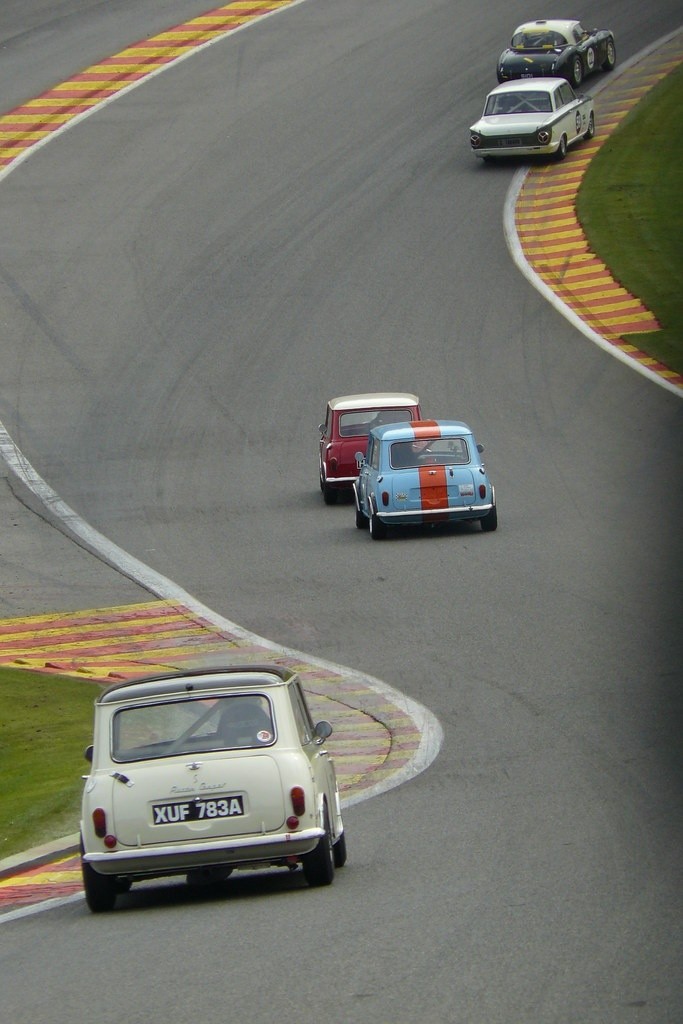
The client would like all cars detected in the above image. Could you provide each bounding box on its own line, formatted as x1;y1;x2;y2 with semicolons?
76;659;348;914
467;76;597;162
495;18;617;88
315;391;429;505
352;418;499;540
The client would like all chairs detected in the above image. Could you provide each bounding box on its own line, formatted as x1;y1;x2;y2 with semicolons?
391;447;416;466
217;704;271;741
